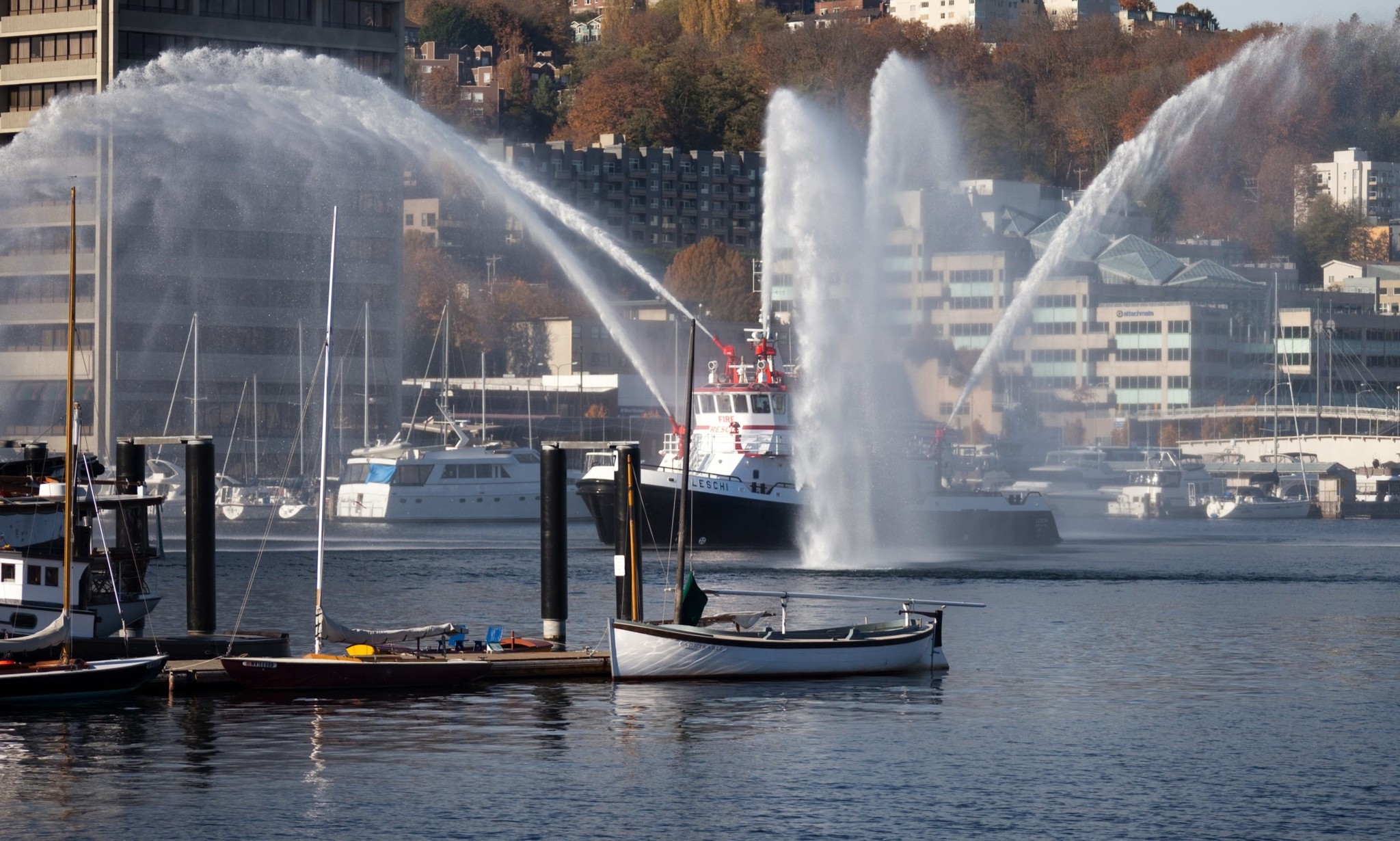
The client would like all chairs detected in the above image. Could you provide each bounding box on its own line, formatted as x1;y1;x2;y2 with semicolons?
472;624;504;653
437;624;469;652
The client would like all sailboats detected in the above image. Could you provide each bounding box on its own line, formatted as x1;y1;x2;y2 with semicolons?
1;187;170;716
217;206;494;699
915;269;1400;522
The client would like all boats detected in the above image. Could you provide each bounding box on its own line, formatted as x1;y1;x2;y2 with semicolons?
574;285;1065;555
0;292;646;658
606;315;986;687
